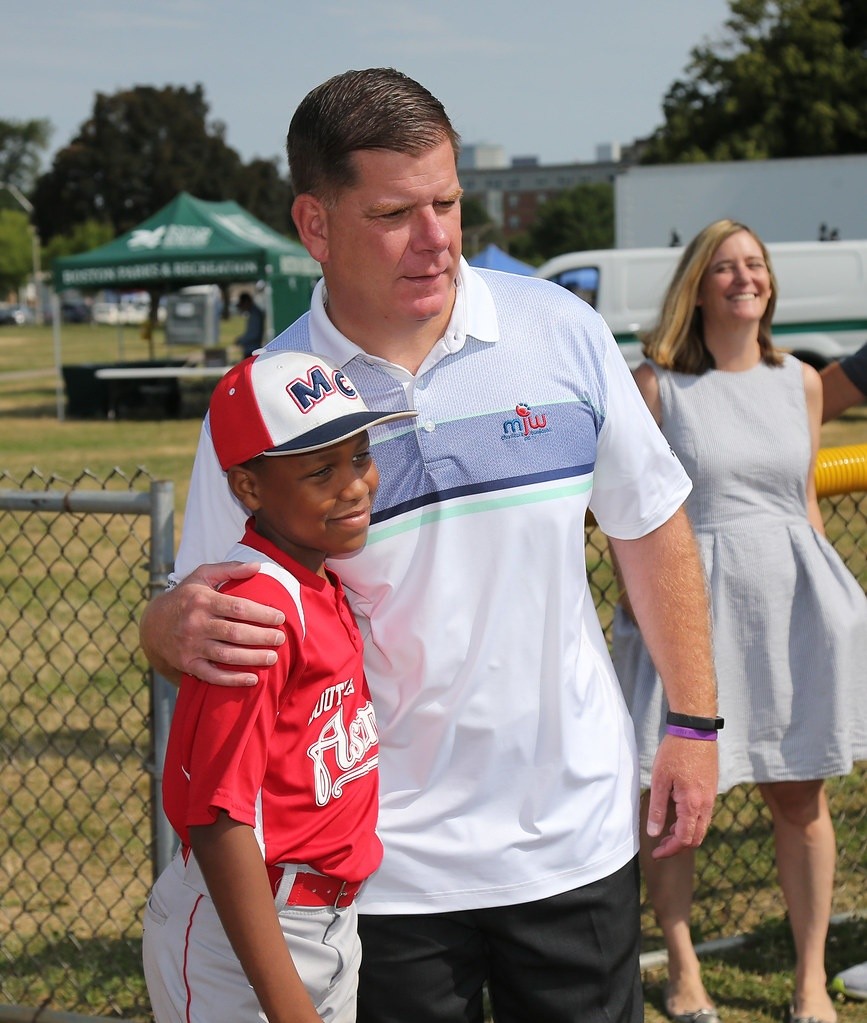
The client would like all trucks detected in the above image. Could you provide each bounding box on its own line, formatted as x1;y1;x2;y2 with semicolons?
531;235;867;374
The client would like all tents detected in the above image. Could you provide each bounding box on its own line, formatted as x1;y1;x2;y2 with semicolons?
467;242;557;282
43;192;324;423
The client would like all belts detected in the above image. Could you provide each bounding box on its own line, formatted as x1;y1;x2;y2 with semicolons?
181;843;364;910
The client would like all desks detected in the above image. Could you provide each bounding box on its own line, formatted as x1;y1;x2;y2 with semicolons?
96;366;234;422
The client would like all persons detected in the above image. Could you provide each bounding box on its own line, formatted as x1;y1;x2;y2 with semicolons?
233;293;265;359
138;66;720;1023
143;345;418;1023
612;217;867;1023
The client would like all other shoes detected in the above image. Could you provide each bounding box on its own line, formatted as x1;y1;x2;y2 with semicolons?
664;987;717;1023
788;1000;832;1023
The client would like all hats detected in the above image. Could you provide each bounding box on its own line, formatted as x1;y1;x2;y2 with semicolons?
210;347;420;471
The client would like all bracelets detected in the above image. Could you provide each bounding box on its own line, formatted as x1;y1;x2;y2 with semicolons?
665;724;717;741
667;711;724;729
